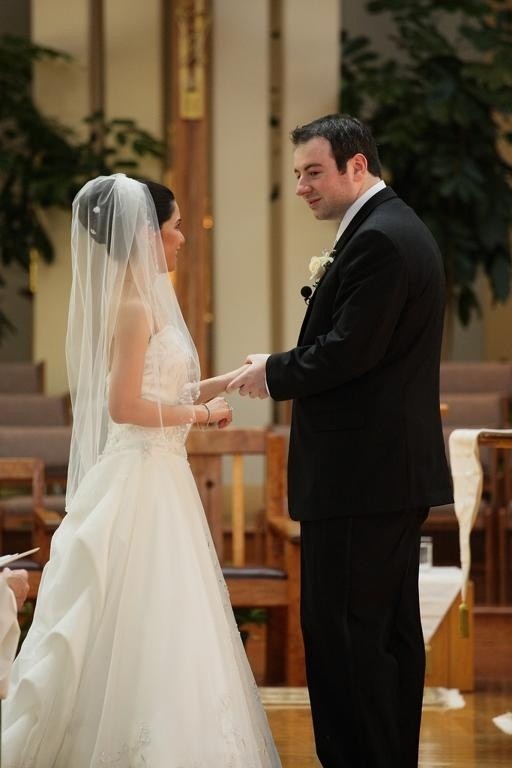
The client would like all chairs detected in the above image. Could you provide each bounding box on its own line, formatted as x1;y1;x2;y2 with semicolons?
0;456;62;658
183;428;301;685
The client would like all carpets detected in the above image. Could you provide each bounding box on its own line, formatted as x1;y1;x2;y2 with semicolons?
259;684;465;710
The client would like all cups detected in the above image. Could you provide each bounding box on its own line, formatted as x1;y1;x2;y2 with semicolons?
418;536;434;569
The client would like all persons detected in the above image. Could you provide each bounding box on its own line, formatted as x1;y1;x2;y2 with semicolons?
227;111;454;768
0;174;283;767
1;560;31;700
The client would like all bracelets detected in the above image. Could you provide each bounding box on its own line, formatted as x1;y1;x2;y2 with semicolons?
200;402;211;428
